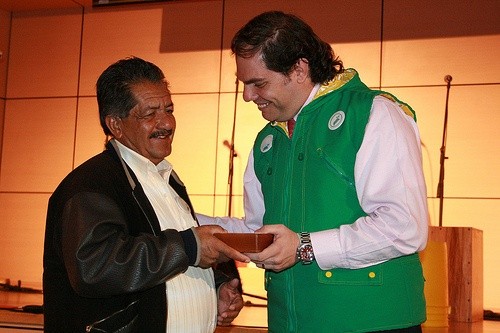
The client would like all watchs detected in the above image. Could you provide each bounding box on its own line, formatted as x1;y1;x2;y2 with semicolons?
294;230;318;268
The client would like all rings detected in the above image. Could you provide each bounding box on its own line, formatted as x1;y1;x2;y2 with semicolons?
262;263;265;269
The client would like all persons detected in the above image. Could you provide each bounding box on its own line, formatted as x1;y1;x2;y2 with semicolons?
193;10;432;332
43;55;254;332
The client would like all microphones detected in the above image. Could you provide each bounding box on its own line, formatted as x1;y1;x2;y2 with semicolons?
444;75;452;83
